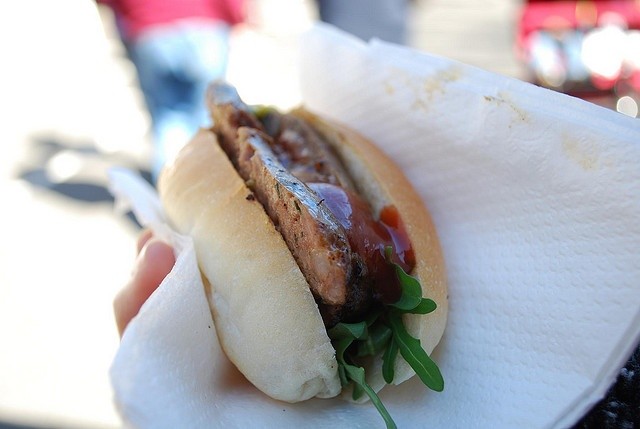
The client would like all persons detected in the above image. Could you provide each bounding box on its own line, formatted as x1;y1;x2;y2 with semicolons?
109;228;177;350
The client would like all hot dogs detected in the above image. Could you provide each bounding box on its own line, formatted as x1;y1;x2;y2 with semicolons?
157;75;450;428
